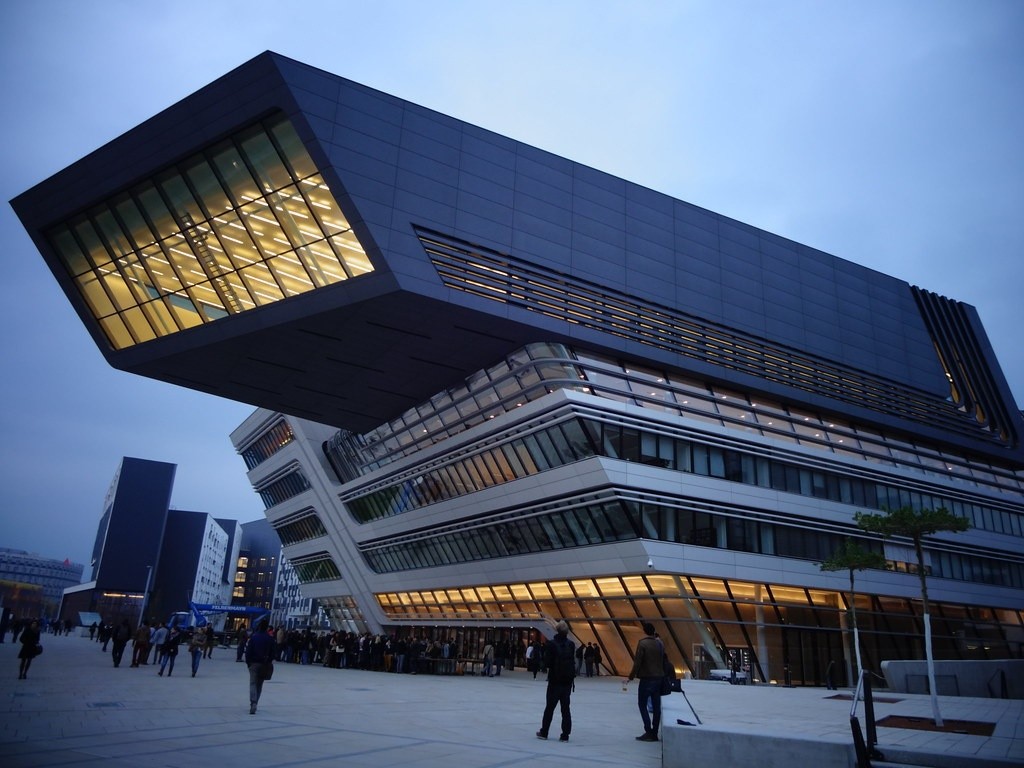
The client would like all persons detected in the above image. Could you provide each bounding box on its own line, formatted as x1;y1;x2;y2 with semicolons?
246;622;272;715
13;617;71;643
269;625;547;679
575;642;601;677
536;621;576;742
89;620;215;678
18;619;40;680
627;623;665;742
236;623;248;662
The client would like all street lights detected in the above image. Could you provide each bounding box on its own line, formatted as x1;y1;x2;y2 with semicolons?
136;563;154;628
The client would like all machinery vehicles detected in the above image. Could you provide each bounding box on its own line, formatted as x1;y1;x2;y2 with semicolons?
168;601;270;647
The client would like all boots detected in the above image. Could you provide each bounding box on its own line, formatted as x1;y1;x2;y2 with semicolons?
158;667;164;676
168;668;172;676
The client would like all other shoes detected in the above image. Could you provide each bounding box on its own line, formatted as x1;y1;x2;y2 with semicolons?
192;672;195;677
18;674;26;679
143;663;149;665
536;732;547;739
208;655;211;659
203;656;205;659
130;663;138;667
236;659;244;662
481;671;484;676
250;704;257;714
560;737;568;742
114;663;119;667
636;731;658;741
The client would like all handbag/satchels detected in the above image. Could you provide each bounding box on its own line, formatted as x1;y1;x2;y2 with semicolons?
33;645;43;655
660;677;671;695
483;655;488;660
255;659;273;680
669;679;681;691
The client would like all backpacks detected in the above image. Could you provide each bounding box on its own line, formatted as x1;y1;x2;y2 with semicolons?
551;640;576;686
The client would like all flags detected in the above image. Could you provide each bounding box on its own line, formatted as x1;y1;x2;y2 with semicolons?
64;559;69;567
